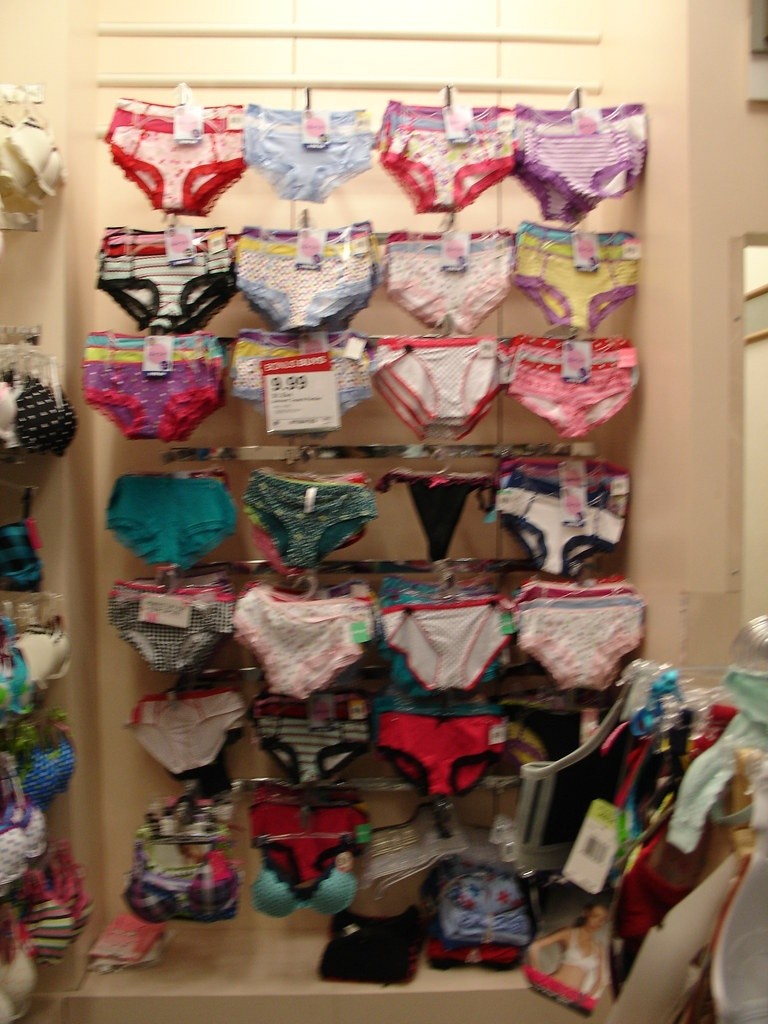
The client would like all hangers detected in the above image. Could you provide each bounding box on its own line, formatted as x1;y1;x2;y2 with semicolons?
0;85;768;896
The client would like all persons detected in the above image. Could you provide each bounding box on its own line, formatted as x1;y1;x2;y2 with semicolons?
525;906;609;1002
